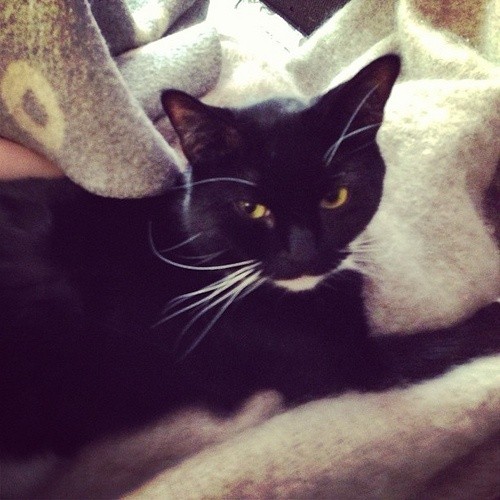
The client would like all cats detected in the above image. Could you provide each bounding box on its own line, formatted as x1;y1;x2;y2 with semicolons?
1;49;500;460
